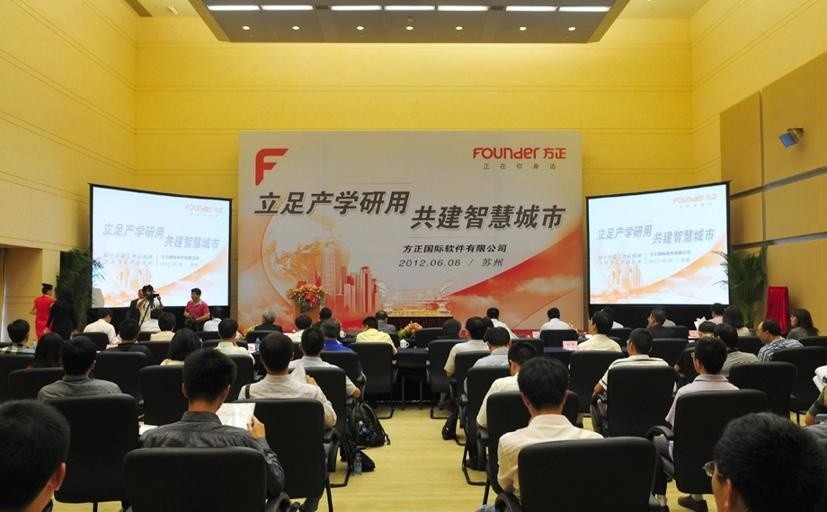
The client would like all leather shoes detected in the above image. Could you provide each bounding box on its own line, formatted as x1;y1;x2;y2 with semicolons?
466;460;477;470
678;495;708;512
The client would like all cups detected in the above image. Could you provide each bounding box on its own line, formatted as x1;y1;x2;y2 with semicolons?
399;338;409;349
339;331;346;338
247;343;255;352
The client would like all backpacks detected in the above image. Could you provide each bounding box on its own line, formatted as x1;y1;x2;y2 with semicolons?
351;401;390;450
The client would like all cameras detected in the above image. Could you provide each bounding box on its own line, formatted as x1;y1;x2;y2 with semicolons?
148;294;159;304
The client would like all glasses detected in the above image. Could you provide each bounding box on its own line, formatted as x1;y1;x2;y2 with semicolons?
702;461;724;478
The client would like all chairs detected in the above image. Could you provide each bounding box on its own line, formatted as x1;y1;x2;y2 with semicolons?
0;327;827;512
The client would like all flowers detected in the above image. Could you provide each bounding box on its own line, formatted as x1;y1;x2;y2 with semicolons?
288;285;328;313
397;322;423;339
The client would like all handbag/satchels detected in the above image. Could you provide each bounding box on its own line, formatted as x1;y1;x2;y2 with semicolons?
346;440;375;473
442;413;458;440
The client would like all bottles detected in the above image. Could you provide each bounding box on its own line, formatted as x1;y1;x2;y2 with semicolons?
353;453;361;476
31;339;37;351
255;338;260;351
292;328;296;335
198;336;203;344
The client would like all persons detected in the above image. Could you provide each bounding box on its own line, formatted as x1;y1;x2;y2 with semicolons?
42;284;80;340
0;320;36;353
139;349;287;487
0;400;69;512
129;287;144;310
805;379;827;460
22;332;122;401
182;287;210;322
576;312;622;352
711;414;827;512
540;307;572;332
82;309;121;345
787;308;820;341
135;285;166;326
647;306;676;337
600;307;623;330
497;358;604;503
30;281;57;341
675;303;803;378
651;336;739;511
151;309;364;429
107;323;152;357
437;308;537;430
594;328;677;416
356;310;398;355
